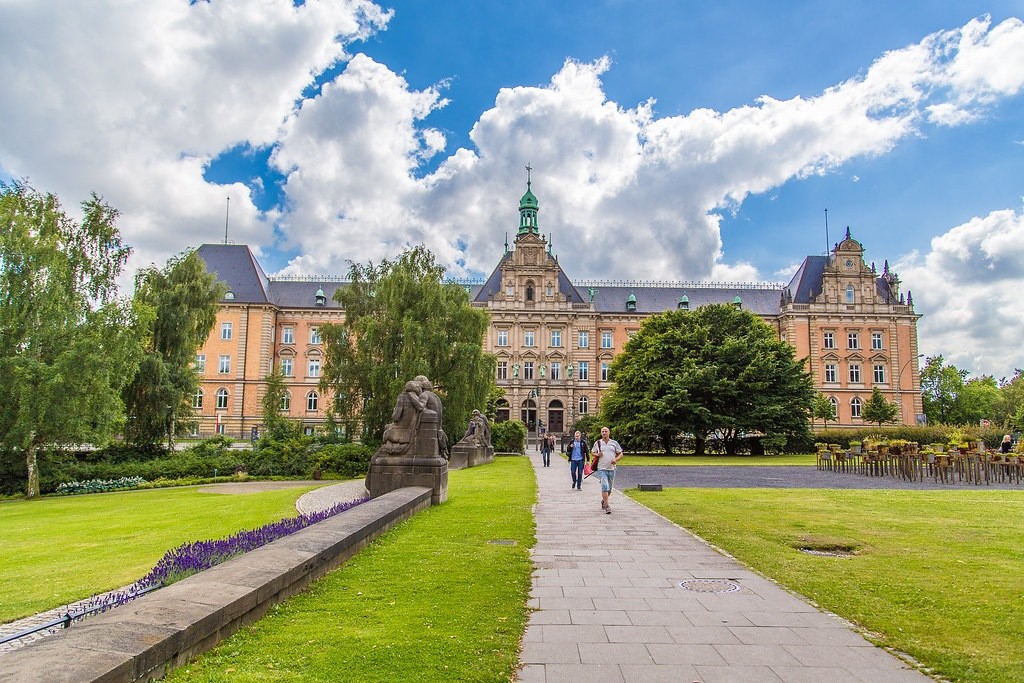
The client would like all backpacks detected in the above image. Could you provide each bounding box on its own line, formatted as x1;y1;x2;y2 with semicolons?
591;440;601;471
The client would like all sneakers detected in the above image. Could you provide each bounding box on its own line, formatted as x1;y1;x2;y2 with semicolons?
601;500;606;509
572;482;576;489
606;505;612;514
577;487;582;491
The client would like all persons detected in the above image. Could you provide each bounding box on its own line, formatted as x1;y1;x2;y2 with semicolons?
566;431;590;491
540;433;556;467
460;409;490;447
383;375;442;457
591;426;623;514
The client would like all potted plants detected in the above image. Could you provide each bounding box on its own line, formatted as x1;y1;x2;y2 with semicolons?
815;438;1024;466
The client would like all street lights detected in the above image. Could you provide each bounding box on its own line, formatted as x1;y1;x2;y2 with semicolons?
895;353;925;426
